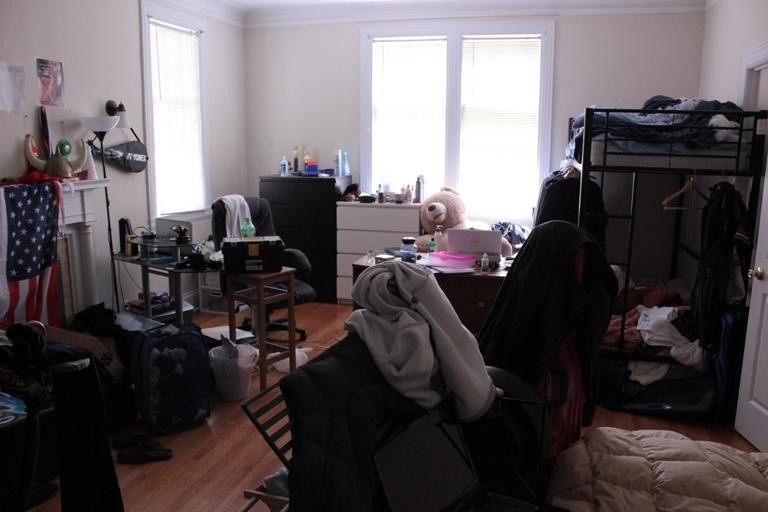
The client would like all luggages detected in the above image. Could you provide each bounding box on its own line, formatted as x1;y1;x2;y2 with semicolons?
130;323;211;436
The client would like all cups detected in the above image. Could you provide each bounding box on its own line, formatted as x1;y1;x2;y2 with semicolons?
378;193;385;203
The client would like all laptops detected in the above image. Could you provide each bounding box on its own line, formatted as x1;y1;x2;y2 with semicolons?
447;229;501;263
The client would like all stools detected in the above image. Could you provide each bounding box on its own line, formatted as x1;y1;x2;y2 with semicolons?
226;266;298;390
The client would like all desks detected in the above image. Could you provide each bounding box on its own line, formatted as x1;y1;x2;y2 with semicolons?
351;250;512;338
112;249;220;333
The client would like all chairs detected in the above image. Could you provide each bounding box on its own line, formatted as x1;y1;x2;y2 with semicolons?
209;196;315;342
242;384;548;512
598;314;744;417
476;219;619;502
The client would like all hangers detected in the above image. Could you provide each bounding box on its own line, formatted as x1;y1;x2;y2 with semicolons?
543;161;598;186
658;169;717;215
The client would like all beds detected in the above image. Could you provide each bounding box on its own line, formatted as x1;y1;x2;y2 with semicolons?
567;109;767;362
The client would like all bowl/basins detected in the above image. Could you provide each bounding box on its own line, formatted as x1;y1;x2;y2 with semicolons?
392;193;406;200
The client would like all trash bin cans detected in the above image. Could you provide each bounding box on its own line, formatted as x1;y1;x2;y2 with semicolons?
208;344;256;400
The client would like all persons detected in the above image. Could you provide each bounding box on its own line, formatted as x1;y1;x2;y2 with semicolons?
40;73;53;103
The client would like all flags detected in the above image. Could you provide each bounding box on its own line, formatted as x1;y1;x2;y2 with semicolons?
0;171;65;330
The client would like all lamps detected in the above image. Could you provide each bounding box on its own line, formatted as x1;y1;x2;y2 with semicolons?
104;100;133;129
82;115;122;314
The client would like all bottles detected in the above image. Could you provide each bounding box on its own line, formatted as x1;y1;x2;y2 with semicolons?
400;227;443;263
279;143;353;177
241;217;256;239
415;176;423;202
481;252;490;274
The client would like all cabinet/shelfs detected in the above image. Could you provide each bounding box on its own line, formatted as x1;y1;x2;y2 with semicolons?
258;177;337;305
336;201;422;305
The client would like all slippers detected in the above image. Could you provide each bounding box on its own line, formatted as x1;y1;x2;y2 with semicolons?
112;435;161;450
118;442;173;464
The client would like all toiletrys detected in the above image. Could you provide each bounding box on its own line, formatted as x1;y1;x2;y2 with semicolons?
333;146;351;176
428;237;437;252
433;227;443;250
280;156;288;176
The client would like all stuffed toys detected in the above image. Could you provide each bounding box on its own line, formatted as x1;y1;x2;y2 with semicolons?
415;185;514;258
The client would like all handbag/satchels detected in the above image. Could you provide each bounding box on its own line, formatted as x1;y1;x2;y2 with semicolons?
708;114;741;142
